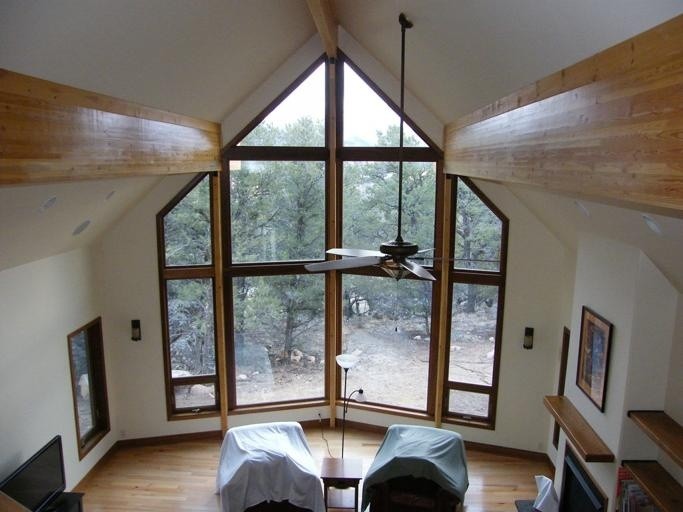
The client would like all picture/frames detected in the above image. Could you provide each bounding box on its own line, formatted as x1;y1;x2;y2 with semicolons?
576;304;614;414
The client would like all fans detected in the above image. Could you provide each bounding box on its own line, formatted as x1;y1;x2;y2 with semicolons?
305;13;500;284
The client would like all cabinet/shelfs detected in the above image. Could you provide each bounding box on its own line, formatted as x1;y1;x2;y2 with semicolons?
40;492;84;511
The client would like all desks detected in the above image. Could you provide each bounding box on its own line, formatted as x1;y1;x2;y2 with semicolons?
319;456;363;511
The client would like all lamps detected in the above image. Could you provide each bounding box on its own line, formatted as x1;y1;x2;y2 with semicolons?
335;352;364;456
